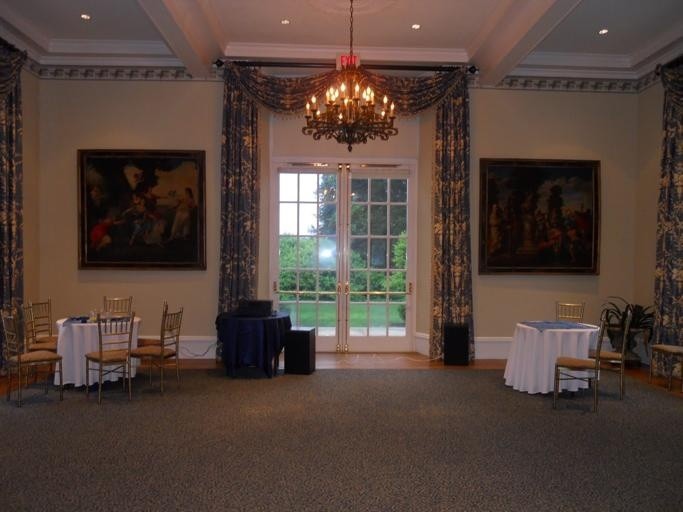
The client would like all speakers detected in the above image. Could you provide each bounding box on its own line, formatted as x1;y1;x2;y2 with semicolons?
283;328;315;373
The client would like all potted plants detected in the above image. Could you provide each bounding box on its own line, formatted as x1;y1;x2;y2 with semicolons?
600;294;656;367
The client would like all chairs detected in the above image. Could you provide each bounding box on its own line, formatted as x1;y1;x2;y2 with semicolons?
2;292;184;402
554;298;632;408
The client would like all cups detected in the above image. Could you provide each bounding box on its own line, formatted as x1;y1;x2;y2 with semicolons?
86;304;106;323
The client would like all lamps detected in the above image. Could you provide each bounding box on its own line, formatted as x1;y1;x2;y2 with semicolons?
302;0;397;146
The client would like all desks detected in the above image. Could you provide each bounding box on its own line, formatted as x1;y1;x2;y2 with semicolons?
215;313;293;378
516;322;600;395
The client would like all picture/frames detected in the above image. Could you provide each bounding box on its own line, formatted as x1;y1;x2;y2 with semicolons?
477;157;601;278
77;148;208;271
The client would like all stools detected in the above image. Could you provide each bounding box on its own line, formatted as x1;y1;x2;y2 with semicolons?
650;344;683;390
